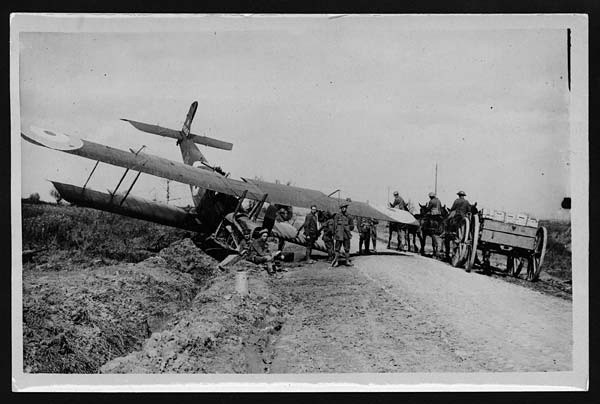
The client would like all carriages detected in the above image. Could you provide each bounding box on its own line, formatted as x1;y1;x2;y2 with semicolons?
388;201;549;281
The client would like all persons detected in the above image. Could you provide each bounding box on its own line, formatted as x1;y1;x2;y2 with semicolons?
248;228;282;274
322;207;336;263
389;191;410;211
357;216;373;253
332;202;355;266
425;191;443;218
220;229;255;271
370;218;379;253
303;205;321;264
438;190;472;238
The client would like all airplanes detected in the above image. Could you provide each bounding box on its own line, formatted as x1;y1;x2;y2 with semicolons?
21;100;421;262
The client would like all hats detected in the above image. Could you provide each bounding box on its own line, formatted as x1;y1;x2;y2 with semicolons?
259;228;269;234
242;229;251;235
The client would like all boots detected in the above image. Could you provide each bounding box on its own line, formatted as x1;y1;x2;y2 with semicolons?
334;252;339;266
345;252;351;265
306;248;313;264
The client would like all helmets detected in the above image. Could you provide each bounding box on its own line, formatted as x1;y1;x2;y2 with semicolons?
339;203;349;209
429;192;436;196
394;191;398;194
456;191;467;196
